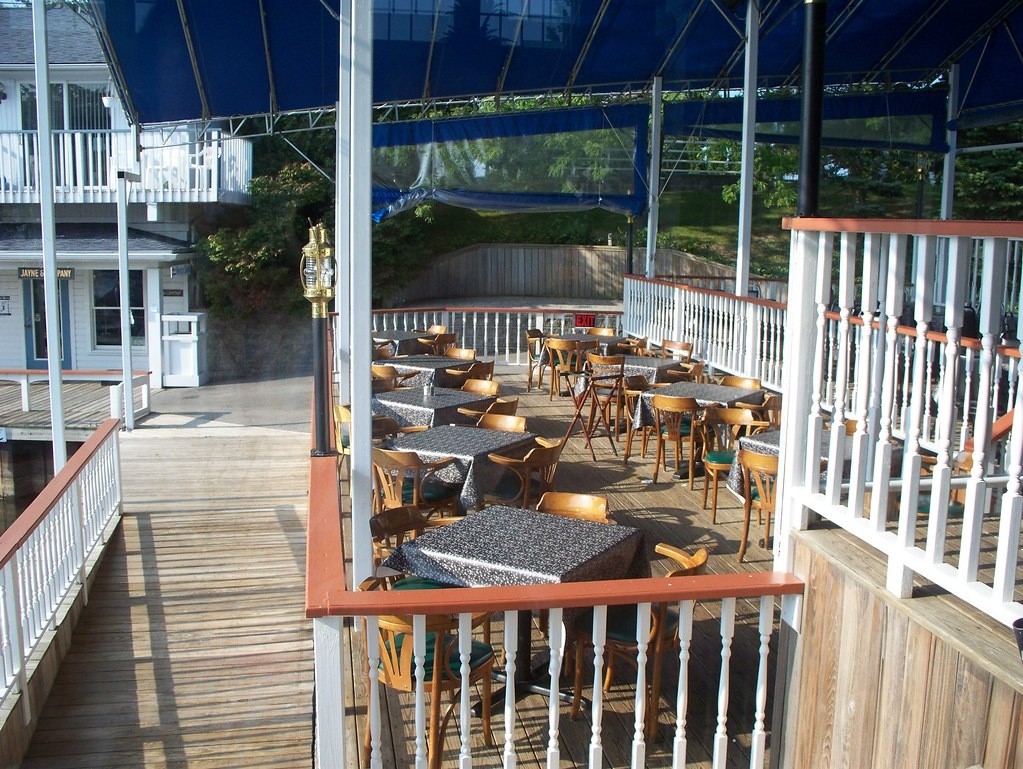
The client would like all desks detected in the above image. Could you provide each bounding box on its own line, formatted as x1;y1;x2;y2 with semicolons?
726;430;903;505
376;505;641;716
372;425;539;509
574;355;684;433
631;381;764;480
371;387;497;428
375;354;476;387
537;334;627;397
372;330;438;356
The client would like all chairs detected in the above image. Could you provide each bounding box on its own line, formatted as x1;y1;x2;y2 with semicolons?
524;327;973;562
334;324;708;769
